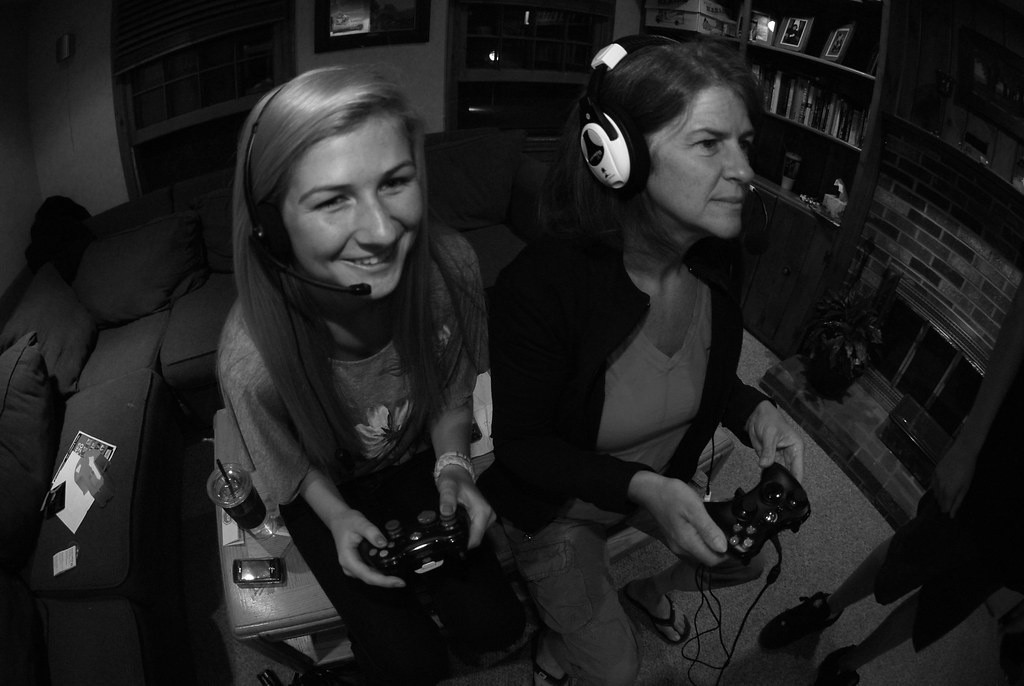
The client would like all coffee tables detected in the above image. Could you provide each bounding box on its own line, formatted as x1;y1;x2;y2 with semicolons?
210;369;737;676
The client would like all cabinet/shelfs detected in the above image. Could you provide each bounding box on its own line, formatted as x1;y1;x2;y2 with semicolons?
111;0;1024;536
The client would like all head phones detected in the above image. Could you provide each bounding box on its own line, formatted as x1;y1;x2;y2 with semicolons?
243;83;295;266
577;33;685;200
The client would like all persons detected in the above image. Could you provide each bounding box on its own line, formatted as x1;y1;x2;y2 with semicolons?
787;25;798;44
489;34;806;686
218;62;498;686
831;34;843;53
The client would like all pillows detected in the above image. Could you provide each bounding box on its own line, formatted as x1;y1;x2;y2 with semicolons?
0;330;53;572
0;262;98;398
422;129;528;230
72;214;180;330
99;209;210;298
184;186;235;273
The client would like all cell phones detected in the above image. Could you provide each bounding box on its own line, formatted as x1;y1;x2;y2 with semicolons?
233;557;282;584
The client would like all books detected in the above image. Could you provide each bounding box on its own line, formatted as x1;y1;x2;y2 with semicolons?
752;61;869;151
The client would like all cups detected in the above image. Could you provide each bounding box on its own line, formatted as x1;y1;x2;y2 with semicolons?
780;152;802;190
206;463;276;539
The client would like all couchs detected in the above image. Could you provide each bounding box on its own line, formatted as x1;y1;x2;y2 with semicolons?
0;124;559;686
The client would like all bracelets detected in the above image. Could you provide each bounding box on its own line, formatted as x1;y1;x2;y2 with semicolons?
433;451;477;486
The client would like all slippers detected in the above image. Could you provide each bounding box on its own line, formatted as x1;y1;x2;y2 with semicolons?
531;627;569;686
624;579;691;647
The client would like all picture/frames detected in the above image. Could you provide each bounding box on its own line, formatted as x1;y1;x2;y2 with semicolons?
314;0;431;53
773;10;815;53
820;19;856;63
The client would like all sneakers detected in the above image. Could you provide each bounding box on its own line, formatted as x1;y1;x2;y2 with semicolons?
758;592;842;652
814;644;860;686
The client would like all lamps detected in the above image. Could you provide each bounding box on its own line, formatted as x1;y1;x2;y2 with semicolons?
56;32;76;61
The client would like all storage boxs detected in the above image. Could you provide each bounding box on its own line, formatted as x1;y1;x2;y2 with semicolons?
644;0;726;35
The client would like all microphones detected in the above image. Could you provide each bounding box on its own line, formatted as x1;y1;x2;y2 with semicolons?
264;253;372;294
